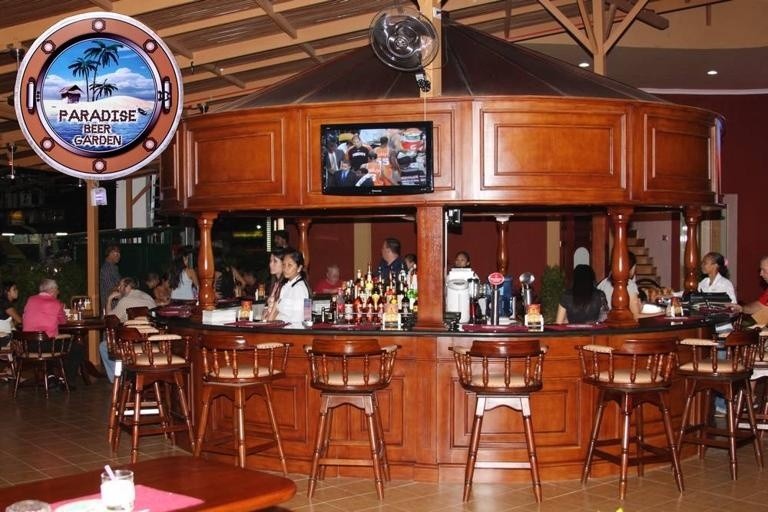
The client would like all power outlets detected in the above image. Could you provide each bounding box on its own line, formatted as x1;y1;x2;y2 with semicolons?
433;7;441;19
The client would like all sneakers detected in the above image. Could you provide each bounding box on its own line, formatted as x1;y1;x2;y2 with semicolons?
742;397;759;412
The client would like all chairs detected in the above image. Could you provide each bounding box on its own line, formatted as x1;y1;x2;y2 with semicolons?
574;336;685;501
12;330;70;400
674;327;765;481
302;338;402;501
193;334;293;480
448;339;549;503
113;326;193;464
105;315;175;438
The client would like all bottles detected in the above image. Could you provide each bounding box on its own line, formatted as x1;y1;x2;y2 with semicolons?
329;296;337;323
413;296;419;318
366;259;374;290
380;278;384;296
355;269;361;285
344;294;353;322
72;303;78;320
402;288;409;320
392;274;396;294
377;293;385;321
336;288;344;318
258;280;265;301
411;264;418;290
348;279;353;295
378;266;382;293
389;265;393;279
353;288;362;321
398;263;407;282
390;295;397;304
344;281;349;301
366;289;375;322
478;282;492;325
672;298;680;317
655;287;663;305
399;277;404;295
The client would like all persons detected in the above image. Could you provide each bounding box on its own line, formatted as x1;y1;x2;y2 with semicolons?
556;264;609;324
275;230;289;248
597;251;666;322
1;281;27;382
98;277;157;383
724;257;768;411
263;249;289;321
274;249;313;329
671;252;736;417
314;264;347;294
404;253;417;273
373;238;408;278
100;244;122;310
455;251;470;268
323;128;427;187
212;251;246;299
23;279;84;391
152;272;171;303
137;272;159;297
638;289;647;313
244;271;260;296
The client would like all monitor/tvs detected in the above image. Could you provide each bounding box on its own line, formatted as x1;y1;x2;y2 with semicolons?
320;121;435;196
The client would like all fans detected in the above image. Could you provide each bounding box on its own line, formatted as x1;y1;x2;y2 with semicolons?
369;5;440;76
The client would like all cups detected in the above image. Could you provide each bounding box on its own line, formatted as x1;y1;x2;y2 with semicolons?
101;472;135;512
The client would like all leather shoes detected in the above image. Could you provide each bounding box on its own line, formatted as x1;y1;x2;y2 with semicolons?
57;376;69;392
48;377;56;388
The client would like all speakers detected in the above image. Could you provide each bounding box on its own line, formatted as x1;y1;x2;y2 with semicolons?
444;209;462;233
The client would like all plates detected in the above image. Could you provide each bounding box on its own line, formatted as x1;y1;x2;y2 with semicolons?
463;325;527;332
148;306;191;317
544;325;609;331
312;323;383;331
224;321;285;329
